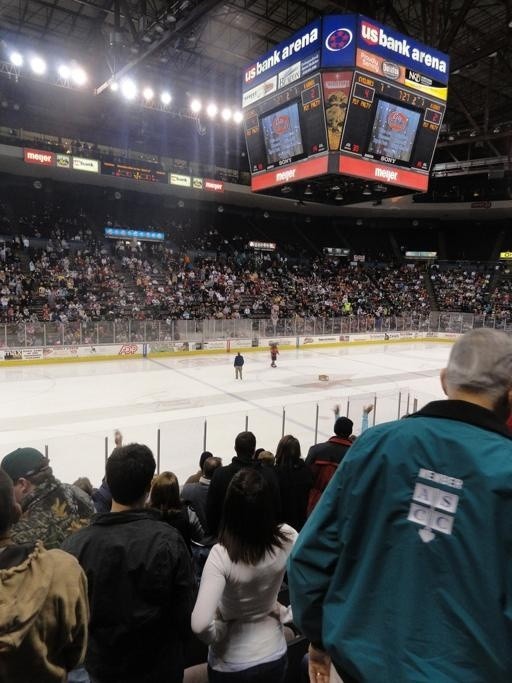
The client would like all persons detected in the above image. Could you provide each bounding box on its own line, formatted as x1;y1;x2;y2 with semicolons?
1;469;90;682
434;259;512;327
2;447;97;549
275;435;313;490
244;240;434;329
270;343;279;367
234;352;244;380
0;205;245;342
180;457;223;538
61;443;198;683
191;469;300;683
257;451;273;473
286;327;511;682
185;452;213;485
71;429;123;513
150;472;207;544
207;431;277;535
305;418;357;516
255;448;264;459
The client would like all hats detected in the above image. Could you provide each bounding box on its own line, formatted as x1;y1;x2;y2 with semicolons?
1;447;49;483
